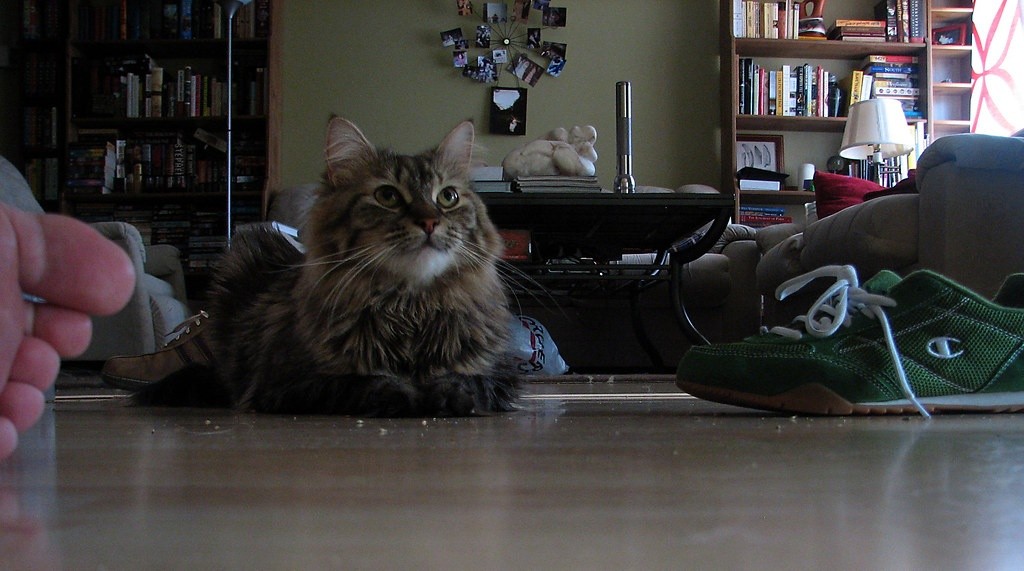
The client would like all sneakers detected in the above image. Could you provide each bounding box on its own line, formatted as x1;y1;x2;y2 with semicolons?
100;310;222;392
675;264;1024;419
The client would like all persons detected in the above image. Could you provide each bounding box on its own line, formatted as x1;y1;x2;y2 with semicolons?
0;201;136;461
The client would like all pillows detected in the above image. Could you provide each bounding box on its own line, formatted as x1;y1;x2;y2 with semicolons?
813;170;886;219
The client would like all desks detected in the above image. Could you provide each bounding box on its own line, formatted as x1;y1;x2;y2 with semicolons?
474;193;734;376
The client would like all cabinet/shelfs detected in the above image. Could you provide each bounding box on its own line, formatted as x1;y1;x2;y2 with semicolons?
717;0;973;226
18;0;282;303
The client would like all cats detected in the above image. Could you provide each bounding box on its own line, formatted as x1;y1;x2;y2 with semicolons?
118;112;565;417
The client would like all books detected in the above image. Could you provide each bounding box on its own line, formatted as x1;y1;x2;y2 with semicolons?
0;0;270;40
874;0;926;44
826;20;886;43
833;121;929;189
22;48;269;277
839;55;922;119
469;176;602;194
733;0;799;40
739;206;791;229
739;58;840;118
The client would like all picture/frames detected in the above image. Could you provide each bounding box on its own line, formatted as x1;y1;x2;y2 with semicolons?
932;23;967;45
736;134;783;190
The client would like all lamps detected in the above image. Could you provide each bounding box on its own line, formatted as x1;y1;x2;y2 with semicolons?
839;98;913;181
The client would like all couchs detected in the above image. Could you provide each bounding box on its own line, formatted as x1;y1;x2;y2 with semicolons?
0;133;1024;424
